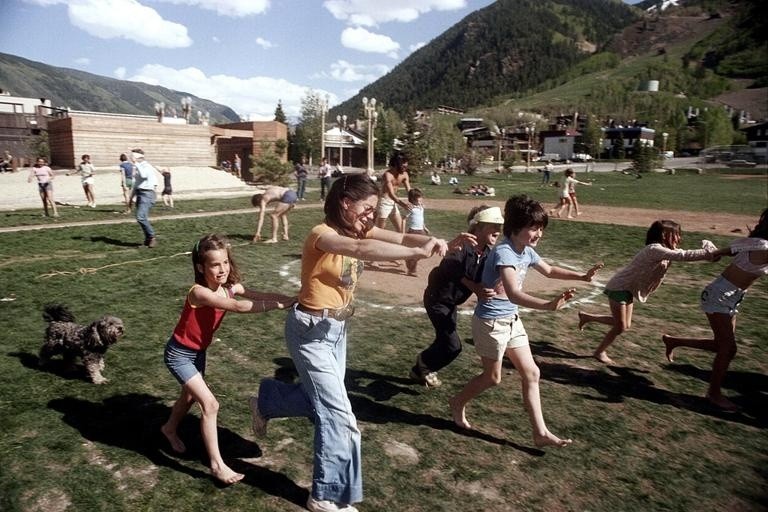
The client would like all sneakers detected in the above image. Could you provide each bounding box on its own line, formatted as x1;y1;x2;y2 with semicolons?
87;202;97;209
248;395;267;440
306;492;359;512
39;212;60;218
136;236;156;248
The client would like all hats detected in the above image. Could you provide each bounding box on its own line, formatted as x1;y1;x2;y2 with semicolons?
469;207;506;230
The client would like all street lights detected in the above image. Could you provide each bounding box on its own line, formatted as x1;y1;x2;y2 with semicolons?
661;132;669;168
316;92;329;167
372;111;377;172
195;110;209;126
362;96;376;174
153;102;165;123
495;128;506;172
179;96;190;123
524;126;535;168
336;114;347;167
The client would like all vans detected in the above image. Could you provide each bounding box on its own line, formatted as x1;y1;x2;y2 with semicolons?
573;152;594;162
542;153;561;162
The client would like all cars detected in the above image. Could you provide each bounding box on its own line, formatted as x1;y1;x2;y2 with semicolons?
727;160;757;169
663;150;673;158
697;151;734;165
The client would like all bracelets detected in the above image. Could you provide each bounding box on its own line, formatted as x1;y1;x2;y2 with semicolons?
262;298;267;312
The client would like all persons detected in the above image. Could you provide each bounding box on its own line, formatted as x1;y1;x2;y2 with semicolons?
663;209;768;411
248;174;479;512
1;147;175;249
543;156;593;220
449;195;604;447
221;153;246;180
578;220;739;365
290;152;346;202
251;185;298;245
431;171;497;198
159;233;298;484
401;188;431;278
409;205;505;387
368;155;414;269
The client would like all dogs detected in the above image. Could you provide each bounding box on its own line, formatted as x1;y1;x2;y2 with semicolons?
36;301;127;385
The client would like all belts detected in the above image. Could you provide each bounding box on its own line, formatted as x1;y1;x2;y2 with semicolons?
297;302;356;321
136;188;153;191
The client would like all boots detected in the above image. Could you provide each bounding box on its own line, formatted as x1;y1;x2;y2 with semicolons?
409;354;442;388
